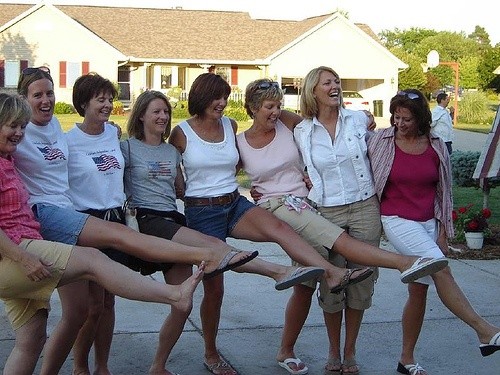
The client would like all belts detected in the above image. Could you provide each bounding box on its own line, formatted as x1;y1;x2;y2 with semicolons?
184;188;240;206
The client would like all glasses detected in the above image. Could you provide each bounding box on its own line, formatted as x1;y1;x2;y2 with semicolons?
22;66;50;81
397;90;420;100
254;82;279;91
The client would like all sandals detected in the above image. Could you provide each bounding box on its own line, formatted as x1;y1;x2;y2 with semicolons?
324;361;342;375
341;360;360;375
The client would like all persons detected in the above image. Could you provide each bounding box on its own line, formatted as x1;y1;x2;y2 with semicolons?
16;67;259;375
0;93;206;375
167;73;376;375
249;66;382;375
65;73;125;375
175;78;449;375
304;90;500;375
430;93;454;155
121;89;325;375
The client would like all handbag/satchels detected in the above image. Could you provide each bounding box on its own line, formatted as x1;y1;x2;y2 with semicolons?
122;207;139;232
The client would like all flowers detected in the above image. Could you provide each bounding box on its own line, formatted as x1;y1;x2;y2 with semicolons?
452;203;495;240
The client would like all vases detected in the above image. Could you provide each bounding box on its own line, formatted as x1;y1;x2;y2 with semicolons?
465;233;483;249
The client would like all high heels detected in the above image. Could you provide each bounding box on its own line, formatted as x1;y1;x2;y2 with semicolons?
479;332;500;357
397;362;428;375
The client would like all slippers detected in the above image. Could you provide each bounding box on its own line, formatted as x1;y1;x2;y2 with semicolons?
275;267;325;290
329;268;374;293
203;360;238;375
203;251;258;279
278;358;308;375
400;256;448;284
